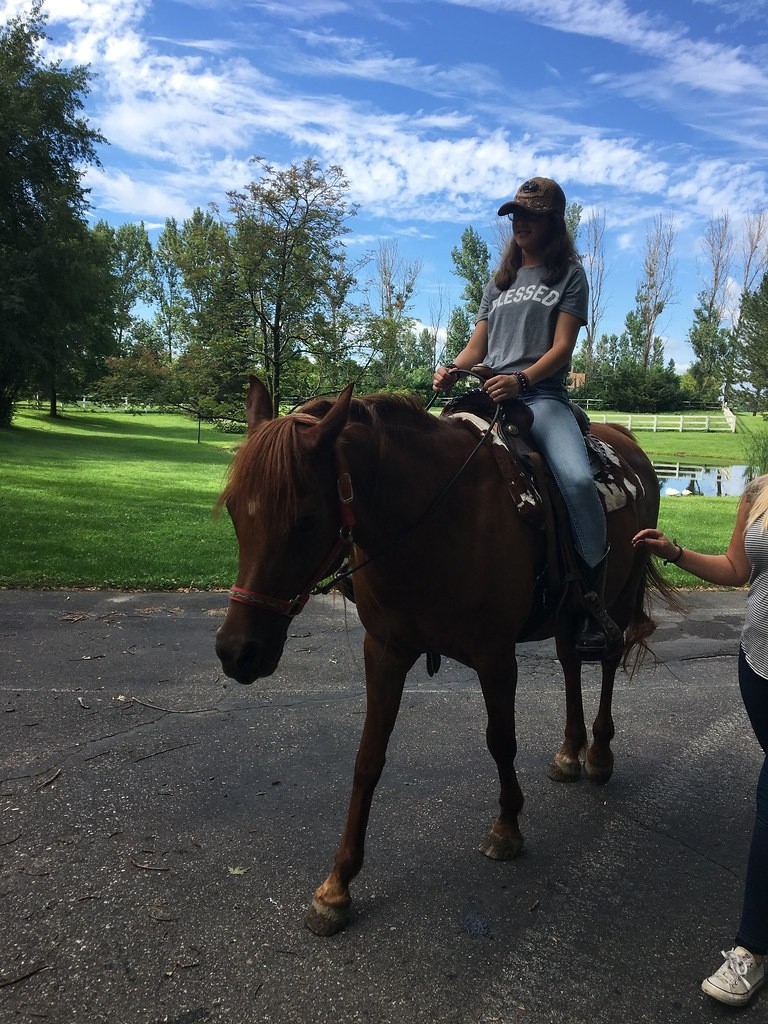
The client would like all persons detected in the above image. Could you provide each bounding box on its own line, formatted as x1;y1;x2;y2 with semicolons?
433;177;610;653
630;472;768;1009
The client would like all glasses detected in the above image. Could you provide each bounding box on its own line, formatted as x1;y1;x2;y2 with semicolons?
507;207;542;221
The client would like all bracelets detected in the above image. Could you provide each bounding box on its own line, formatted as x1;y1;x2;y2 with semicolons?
513;371;530;396
664;538;683;566
446;364;460;385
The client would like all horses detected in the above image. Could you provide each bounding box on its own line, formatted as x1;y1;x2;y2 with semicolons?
211;374;701;936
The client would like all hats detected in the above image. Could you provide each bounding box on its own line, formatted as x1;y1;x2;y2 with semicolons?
497;177;566;220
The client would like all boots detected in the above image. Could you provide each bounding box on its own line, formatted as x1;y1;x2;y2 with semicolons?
574;542;610;658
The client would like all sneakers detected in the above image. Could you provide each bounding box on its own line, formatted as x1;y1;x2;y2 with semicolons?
701;946;765;1006
